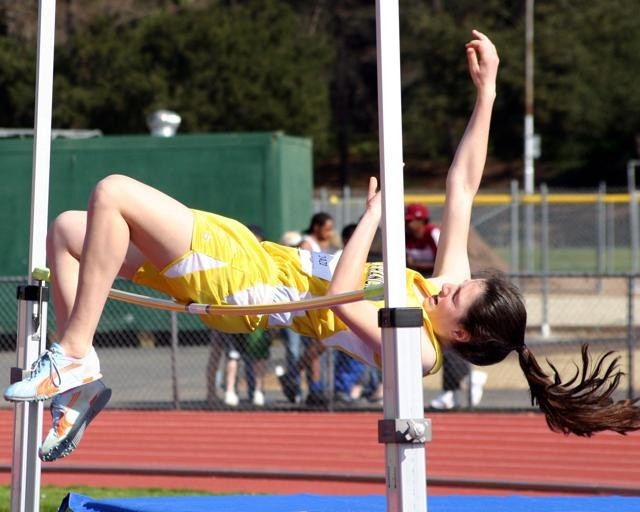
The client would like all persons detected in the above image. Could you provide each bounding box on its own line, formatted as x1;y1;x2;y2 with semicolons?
205;202;489;411
3;29;639;462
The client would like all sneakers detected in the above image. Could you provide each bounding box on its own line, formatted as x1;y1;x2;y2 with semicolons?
38;380;112;463
3;340;105;404
222;389;269;407
430;395;458;410
466;369;488;409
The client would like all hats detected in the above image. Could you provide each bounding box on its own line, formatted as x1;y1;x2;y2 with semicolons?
281;231;302;247
405;203;430;221
